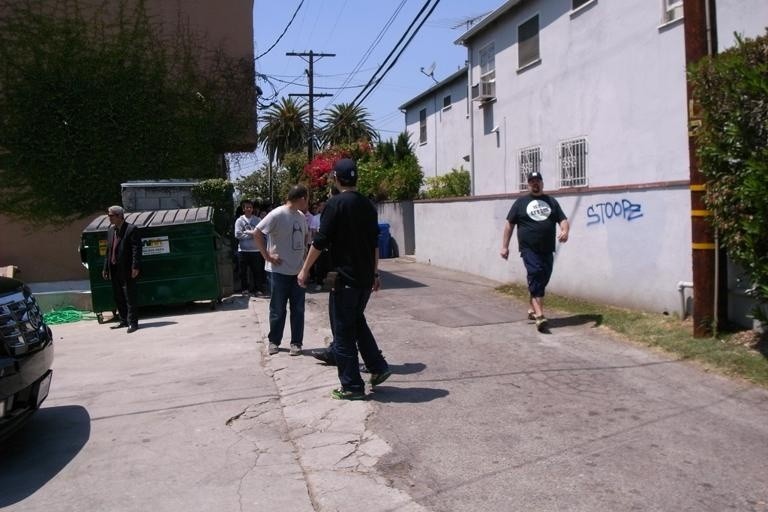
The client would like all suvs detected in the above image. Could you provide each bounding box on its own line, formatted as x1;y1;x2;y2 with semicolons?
0;276;53;442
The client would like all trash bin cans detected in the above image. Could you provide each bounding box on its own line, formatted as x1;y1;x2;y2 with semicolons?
80;206;222;324
376;223;392;258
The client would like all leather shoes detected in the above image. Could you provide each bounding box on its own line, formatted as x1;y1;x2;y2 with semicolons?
110;322;128;329
127;324;139;333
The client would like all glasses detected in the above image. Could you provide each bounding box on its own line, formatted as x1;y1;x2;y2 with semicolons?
107;213;118;217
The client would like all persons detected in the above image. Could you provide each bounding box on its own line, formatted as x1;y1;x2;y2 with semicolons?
500;171;570;331
101;205;143;333
229;158;393;400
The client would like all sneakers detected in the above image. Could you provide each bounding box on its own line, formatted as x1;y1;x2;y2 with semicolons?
368;368;392;385
289;343;302;356
331;386;366;401
527;311;538;324
534;315;550;332
268;341;278;354
311;347;337;365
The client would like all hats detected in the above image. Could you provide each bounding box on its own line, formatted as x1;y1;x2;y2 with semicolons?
333;159;358;181
527;171;542;182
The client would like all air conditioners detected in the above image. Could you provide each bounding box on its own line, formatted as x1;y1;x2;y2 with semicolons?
471;81;495;101
